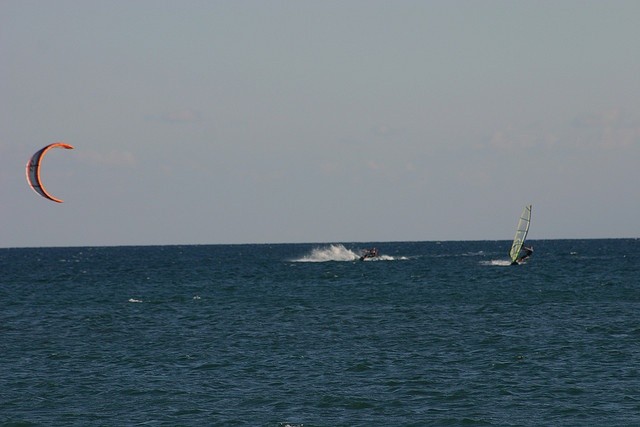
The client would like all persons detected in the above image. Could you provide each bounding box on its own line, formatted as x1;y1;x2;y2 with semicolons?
519;245;535;264
